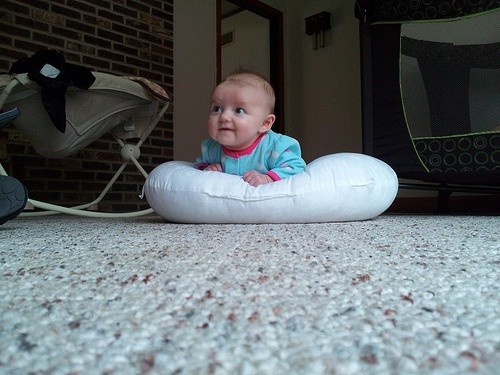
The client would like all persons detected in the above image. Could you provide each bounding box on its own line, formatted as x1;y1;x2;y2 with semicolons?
192;67;307;187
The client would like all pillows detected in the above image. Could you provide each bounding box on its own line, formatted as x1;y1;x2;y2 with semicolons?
139;153;399;224
